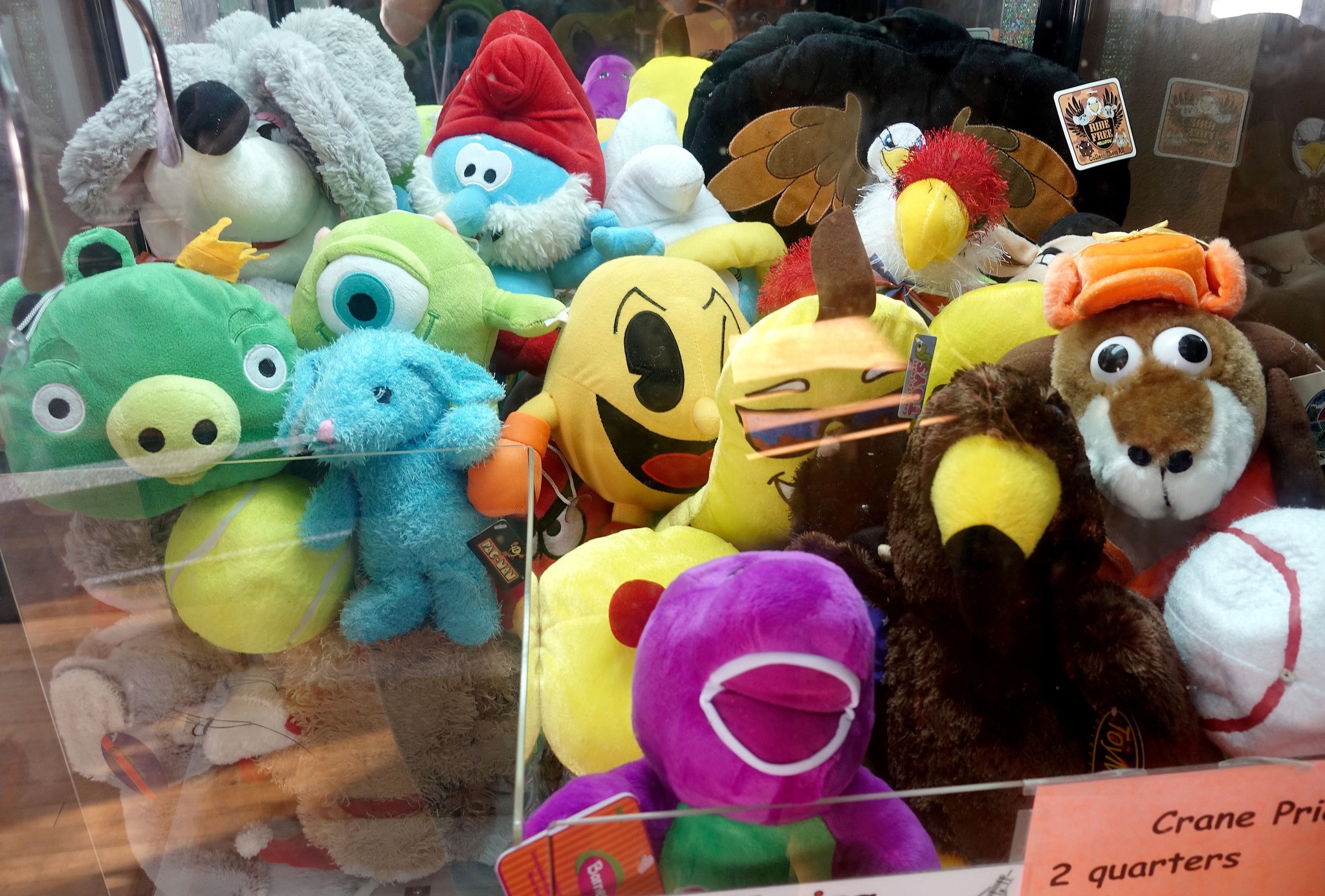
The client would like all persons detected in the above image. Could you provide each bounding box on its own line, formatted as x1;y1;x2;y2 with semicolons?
377;0;701;105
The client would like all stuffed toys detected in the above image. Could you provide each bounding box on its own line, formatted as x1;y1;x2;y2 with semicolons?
3;7;1325;896
